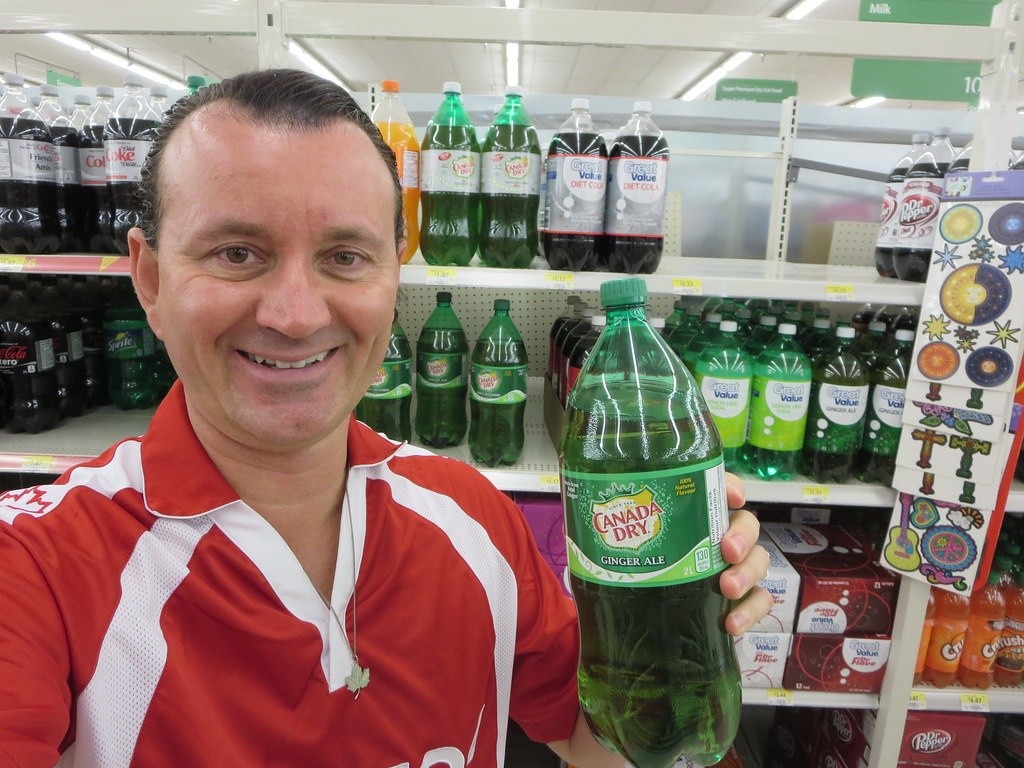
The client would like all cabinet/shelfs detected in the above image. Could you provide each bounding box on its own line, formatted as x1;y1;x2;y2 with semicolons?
0;97;1023;767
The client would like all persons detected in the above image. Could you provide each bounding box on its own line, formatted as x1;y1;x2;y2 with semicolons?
0;69;773;768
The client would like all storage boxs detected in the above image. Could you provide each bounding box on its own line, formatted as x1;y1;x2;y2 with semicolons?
504;489;573;600
761;520;899;632
742;522;800;632
784;631;891;694
669;701;1024;767
732;632;791;687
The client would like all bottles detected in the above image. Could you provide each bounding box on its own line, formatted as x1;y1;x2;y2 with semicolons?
369;77;420;262
547;279;914;768
541;99;608;273
606;101;669;274
915;514;1024;689
0;74;209;253
0;274;180;434
354;308;412;443
415;292;469;448
469;300;529;467
419;82;480;266
480;86;542;267
875;126;1024;283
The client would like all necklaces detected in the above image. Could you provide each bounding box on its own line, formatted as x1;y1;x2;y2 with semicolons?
314;472;370;698
